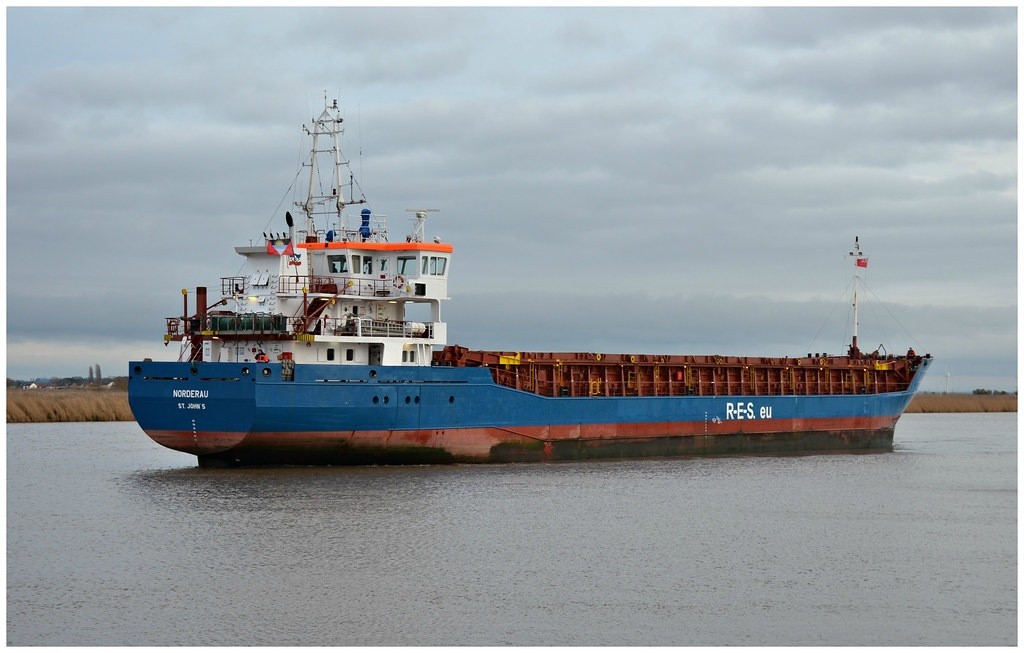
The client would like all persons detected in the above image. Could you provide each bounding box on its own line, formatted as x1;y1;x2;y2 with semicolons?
255;349;269;363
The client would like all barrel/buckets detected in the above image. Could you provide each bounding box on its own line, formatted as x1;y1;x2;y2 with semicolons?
405;322;426;334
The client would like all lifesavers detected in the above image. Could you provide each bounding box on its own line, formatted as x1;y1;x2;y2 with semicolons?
393;276;404;289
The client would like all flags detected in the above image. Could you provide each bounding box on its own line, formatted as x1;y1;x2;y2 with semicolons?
267;239;293;255
289;253;301;265
857;258;867;267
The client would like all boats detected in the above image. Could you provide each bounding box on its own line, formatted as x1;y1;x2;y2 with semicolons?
126;92;935;467
